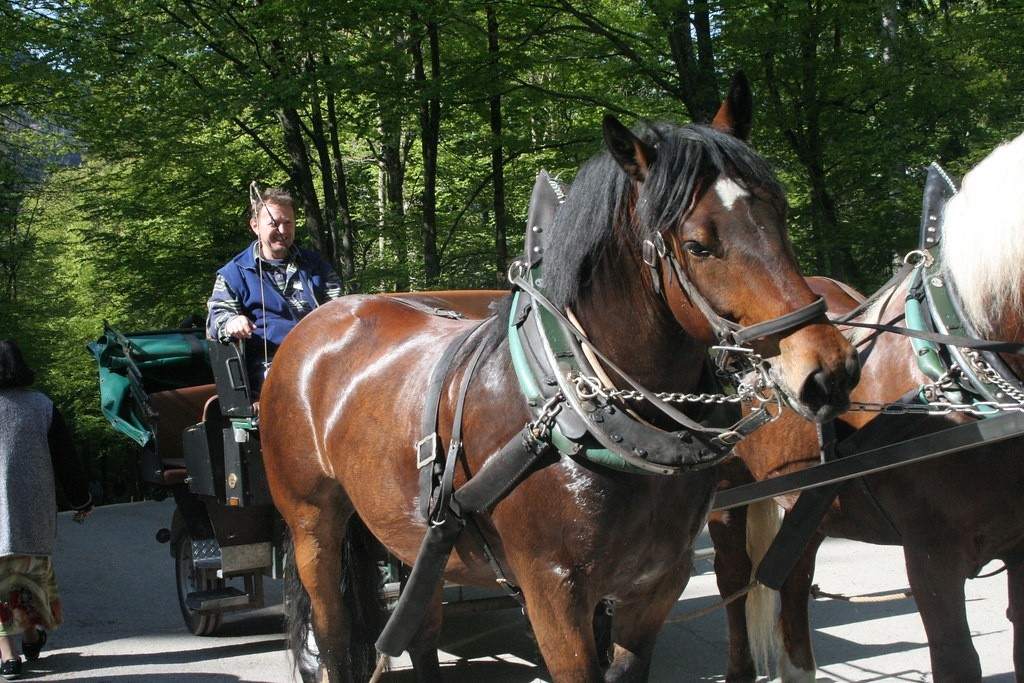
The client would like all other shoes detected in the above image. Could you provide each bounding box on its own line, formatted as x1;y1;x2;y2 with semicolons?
0;656;22;678
22;627;48;659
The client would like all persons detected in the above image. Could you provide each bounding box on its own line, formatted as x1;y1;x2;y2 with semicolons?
0;340;96;680
204;187;346;392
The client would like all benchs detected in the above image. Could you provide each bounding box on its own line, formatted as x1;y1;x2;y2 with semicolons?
133;384;218;485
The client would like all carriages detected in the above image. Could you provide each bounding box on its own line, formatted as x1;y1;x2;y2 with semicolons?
82;69;1024;682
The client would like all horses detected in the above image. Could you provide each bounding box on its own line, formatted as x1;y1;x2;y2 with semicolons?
258;68;1024;683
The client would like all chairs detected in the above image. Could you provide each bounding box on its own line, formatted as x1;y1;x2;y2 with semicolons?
207;333;259;417
